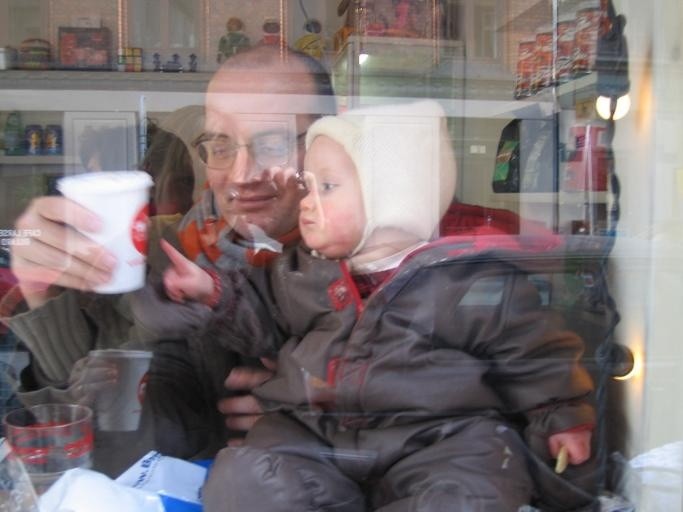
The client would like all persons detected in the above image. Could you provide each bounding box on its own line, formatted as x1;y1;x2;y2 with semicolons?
2;43;596;511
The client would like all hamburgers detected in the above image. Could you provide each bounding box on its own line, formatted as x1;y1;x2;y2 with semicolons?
20;39;51;69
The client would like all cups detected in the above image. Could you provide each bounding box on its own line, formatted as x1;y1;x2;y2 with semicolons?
88;348;153;433
2;402;95;494
56;171;155;297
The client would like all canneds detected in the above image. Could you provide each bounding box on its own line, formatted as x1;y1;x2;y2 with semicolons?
43;125;63;155
514;0;600;99
24;125;43;155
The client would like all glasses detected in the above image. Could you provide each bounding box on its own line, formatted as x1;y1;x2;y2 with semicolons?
190;129;307;172
